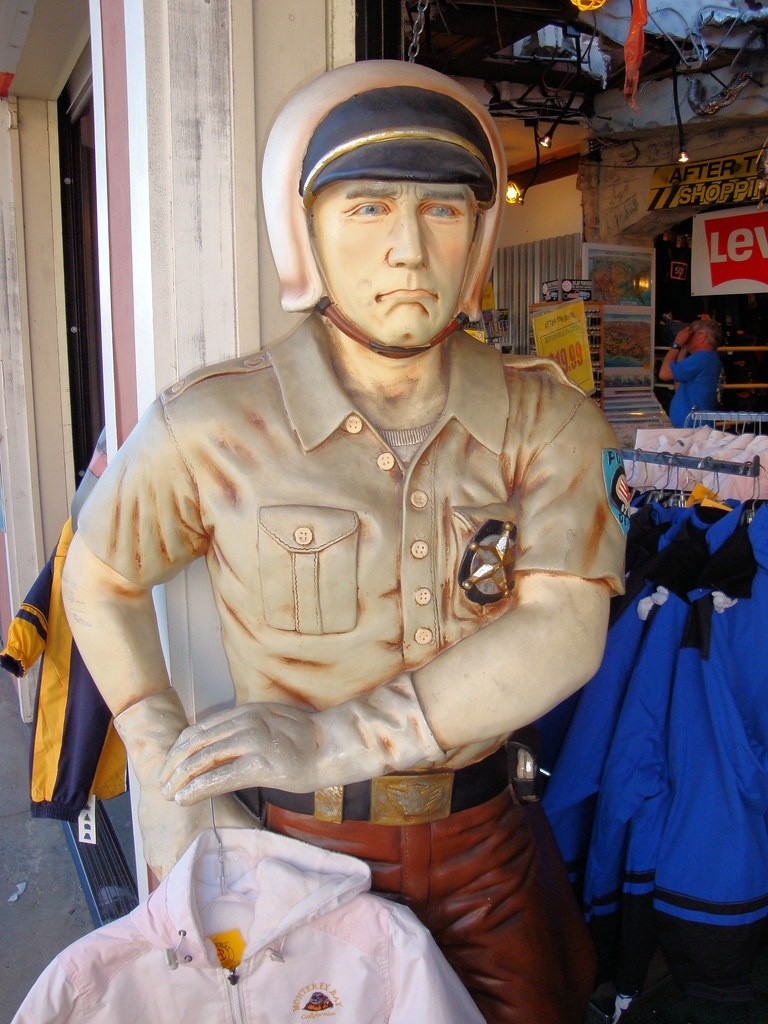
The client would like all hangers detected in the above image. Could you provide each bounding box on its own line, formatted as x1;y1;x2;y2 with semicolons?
620;411;768;528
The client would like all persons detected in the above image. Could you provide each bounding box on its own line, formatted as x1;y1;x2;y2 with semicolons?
660;319;723;430
57;61;632;1023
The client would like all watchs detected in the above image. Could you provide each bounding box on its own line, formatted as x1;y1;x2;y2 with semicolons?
671;343;681;352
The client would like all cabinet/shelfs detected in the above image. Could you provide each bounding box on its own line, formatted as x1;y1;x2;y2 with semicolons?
527;300;603;411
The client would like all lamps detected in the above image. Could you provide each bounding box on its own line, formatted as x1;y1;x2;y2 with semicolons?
672;50;690;163
504;120;540;206
537;23;582;148
